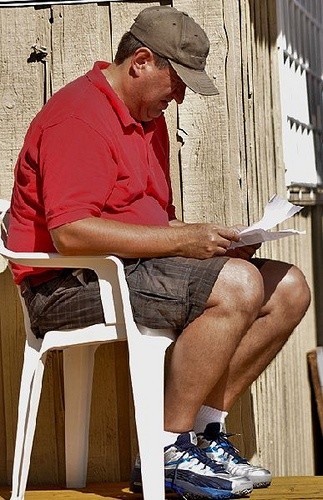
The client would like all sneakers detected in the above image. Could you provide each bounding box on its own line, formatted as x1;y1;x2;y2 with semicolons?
130;430;254;500
193;423;272;488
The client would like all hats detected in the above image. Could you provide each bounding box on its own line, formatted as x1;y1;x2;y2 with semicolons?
130;6;220;96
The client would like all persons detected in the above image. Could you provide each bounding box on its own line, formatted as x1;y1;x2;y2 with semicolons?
6;5;312;500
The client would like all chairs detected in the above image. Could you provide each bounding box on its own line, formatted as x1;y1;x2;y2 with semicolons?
0;199;228;500
